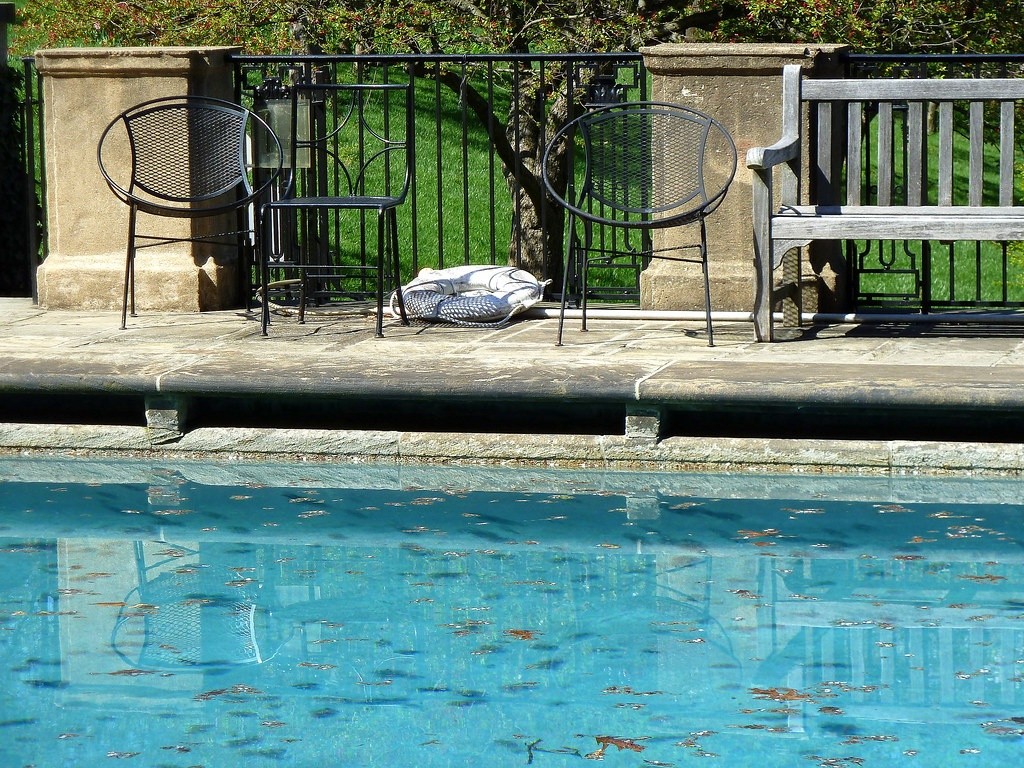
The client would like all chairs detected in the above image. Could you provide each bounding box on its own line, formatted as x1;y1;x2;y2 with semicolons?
96;95;284;332
542;101;737;347
258;81;416;336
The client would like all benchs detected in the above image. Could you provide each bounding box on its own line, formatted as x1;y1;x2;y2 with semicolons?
746;65;1024;343
